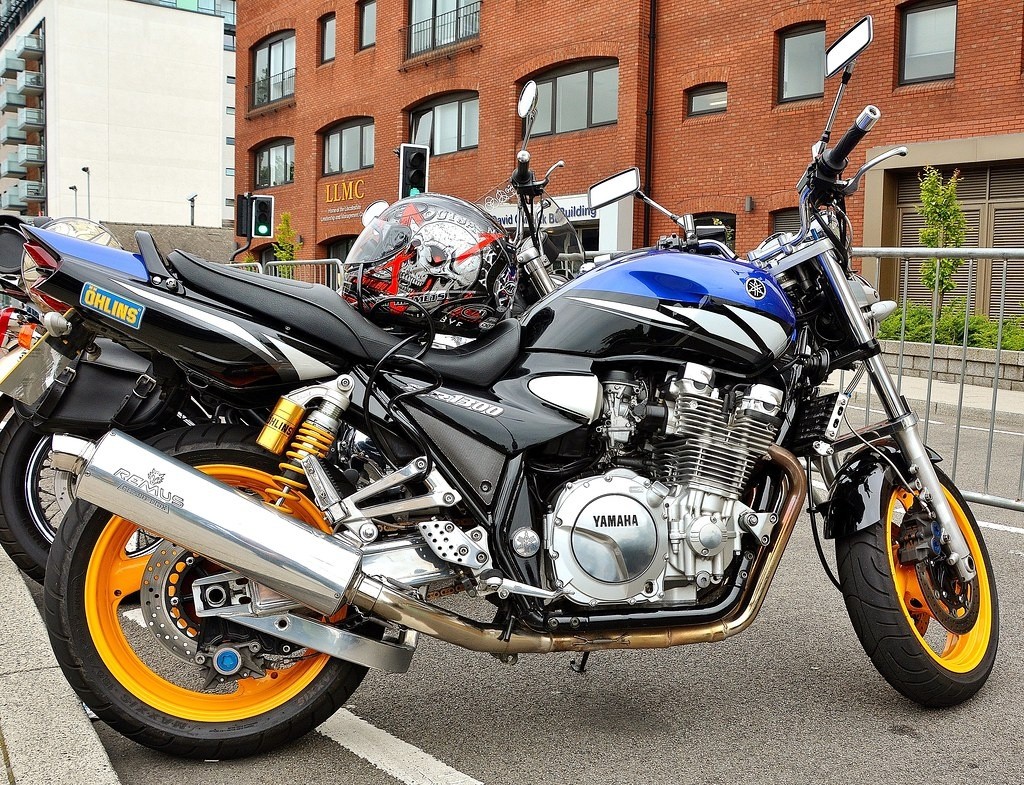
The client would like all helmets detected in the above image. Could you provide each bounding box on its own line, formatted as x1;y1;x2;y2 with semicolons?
341;193;518;338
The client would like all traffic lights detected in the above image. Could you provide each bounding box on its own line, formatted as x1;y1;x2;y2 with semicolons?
252;195;274;238
399;143;430;200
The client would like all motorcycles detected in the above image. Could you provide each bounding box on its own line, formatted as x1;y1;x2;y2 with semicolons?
0;15;999;762
